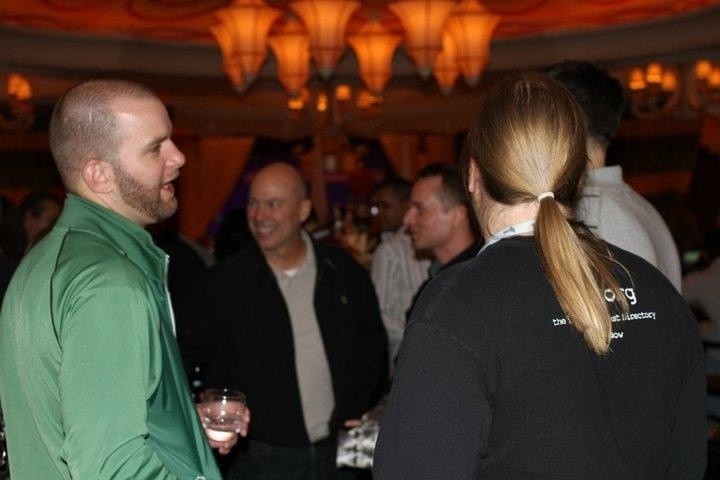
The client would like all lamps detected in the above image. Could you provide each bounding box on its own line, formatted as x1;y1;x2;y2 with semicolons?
625;57;720;122
0;72;34;132
208;0;503;112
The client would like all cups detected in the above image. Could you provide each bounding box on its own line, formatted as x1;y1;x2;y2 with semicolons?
200;388;247;447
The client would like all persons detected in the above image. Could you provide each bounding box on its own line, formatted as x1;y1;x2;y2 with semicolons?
544;60;686;303
0;76;252;476
373;68;718;476
1;91;718;480
